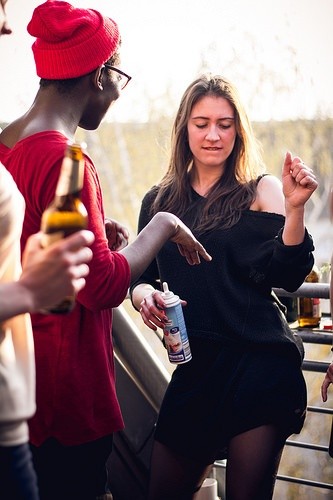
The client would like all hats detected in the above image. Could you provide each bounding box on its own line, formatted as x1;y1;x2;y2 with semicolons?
27;0;119;80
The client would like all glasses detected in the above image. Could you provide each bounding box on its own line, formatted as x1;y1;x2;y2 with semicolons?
105;64;132;90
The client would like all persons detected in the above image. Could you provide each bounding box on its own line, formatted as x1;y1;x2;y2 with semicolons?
0;0;213;500
0;0;95;500
130;76;318;500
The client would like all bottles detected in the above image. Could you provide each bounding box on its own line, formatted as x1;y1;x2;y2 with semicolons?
297;269;321;328
157;282;192;365
38;143;88;315
